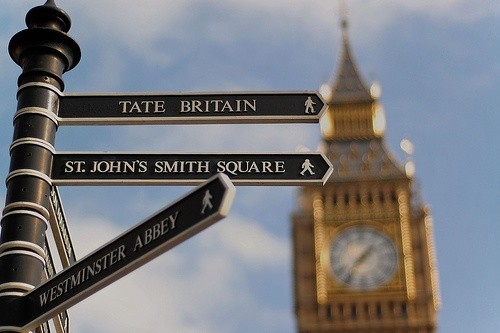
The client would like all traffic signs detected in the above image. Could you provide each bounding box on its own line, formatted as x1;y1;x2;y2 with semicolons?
19;173;236;331
48;185;77;269
56;92;330;123
42;319;50;333
52;151;334;186
40;232;70;333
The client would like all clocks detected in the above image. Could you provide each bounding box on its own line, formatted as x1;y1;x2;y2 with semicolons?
329;228;398;290
